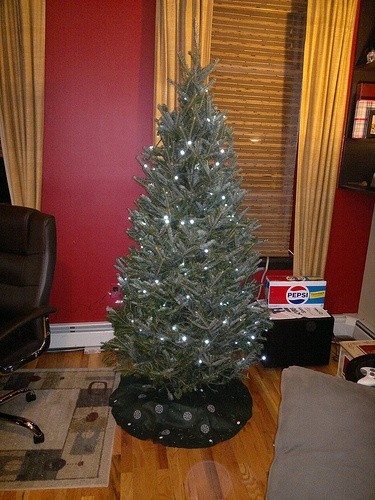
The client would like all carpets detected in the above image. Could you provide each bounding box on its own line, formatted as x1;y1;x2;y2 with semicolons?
0;369;120;491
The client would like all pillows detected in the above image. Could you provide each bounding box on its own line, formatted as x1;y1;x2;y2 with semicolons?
266;366;375;500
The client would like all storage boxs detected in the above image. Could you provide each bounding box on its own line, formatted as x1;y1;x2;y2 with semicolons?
265;275;326;310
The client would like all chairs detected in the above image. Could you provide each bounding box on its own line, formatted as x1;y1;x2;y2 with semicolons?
0;206;58;445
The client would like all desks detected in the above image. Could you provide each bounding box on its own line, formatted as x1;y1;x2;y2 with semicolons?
256;302;334;369
338;340;375;379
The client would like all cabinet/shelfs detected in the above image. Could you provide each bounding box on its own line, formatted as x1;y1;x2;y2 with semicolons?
338;0;375;193
